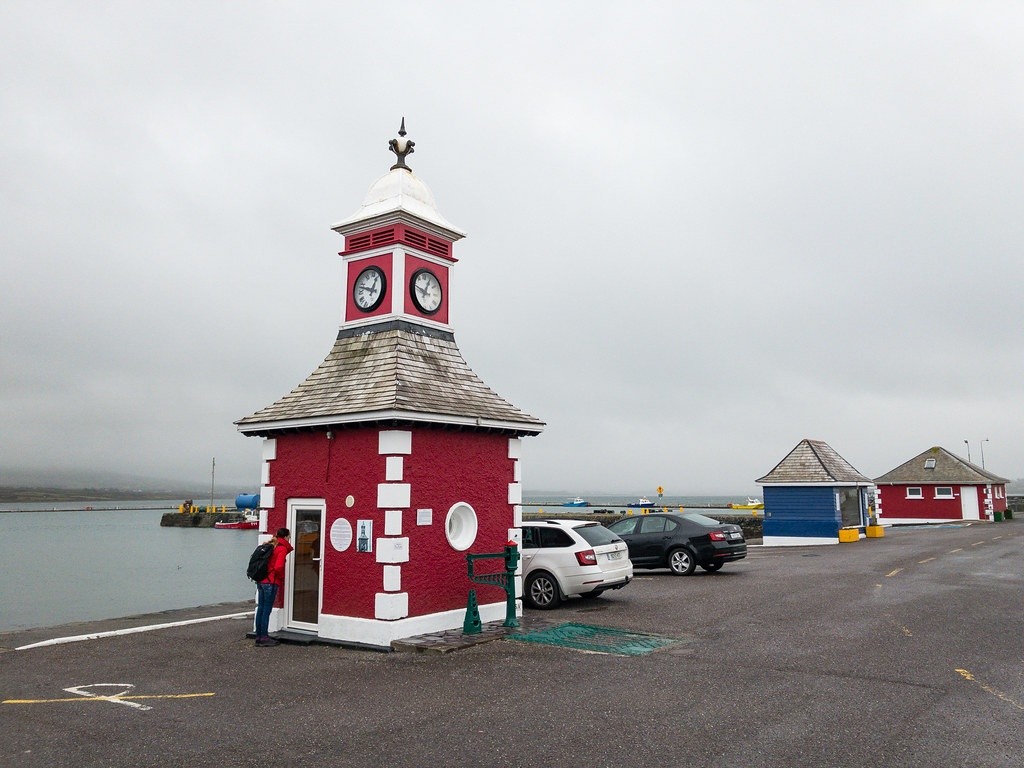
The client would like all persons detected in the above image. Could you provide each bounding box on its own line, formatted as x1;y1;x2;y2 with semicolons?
254;527;294;647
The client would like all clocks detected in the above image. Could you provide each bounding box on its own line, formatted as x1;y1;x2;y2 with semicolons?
353;264;387;313
409;268;443;316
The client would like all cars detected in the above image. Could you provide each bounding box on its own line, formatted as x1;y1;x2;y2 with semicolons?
605;512;747;575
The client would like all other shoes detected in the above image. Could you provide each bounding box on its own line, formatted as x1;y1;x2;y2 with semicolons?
255;638;280;647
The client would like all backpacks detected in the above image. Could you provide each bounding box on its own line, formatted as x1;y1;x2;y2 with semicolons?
247;540;282;582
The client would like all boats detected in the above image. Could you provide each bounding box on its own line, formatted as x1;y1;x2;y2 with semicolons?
732;497;764;509
214;509;260;529
627;496;655;507
562;497;588;507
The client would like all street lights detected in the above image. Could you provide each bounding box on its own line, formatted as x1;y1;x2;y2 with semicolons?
965;440;970;463
981;439;989;469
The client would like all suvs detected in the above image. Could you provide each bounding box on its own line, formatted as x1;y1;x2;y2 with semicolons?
522;520;634;608
